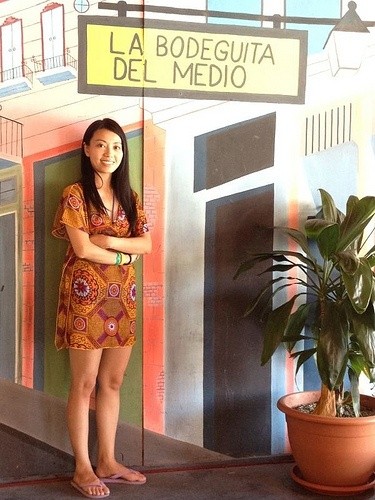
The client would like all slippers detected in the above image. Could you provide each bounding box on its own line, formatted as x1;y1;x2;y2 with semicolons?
99;468;146;485
70;480;109;498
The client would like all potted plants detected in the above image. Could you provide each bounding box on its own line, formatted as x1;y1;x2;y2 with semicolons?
232;189;375;488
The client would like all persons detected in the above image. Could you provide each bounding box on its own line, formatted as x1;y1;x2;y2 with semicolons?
50;118;152;499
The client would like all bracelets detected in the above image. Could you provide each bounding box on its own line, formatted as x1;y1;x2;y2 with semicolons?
125;254;132;265
119;253;126;265
115;253;122;266
